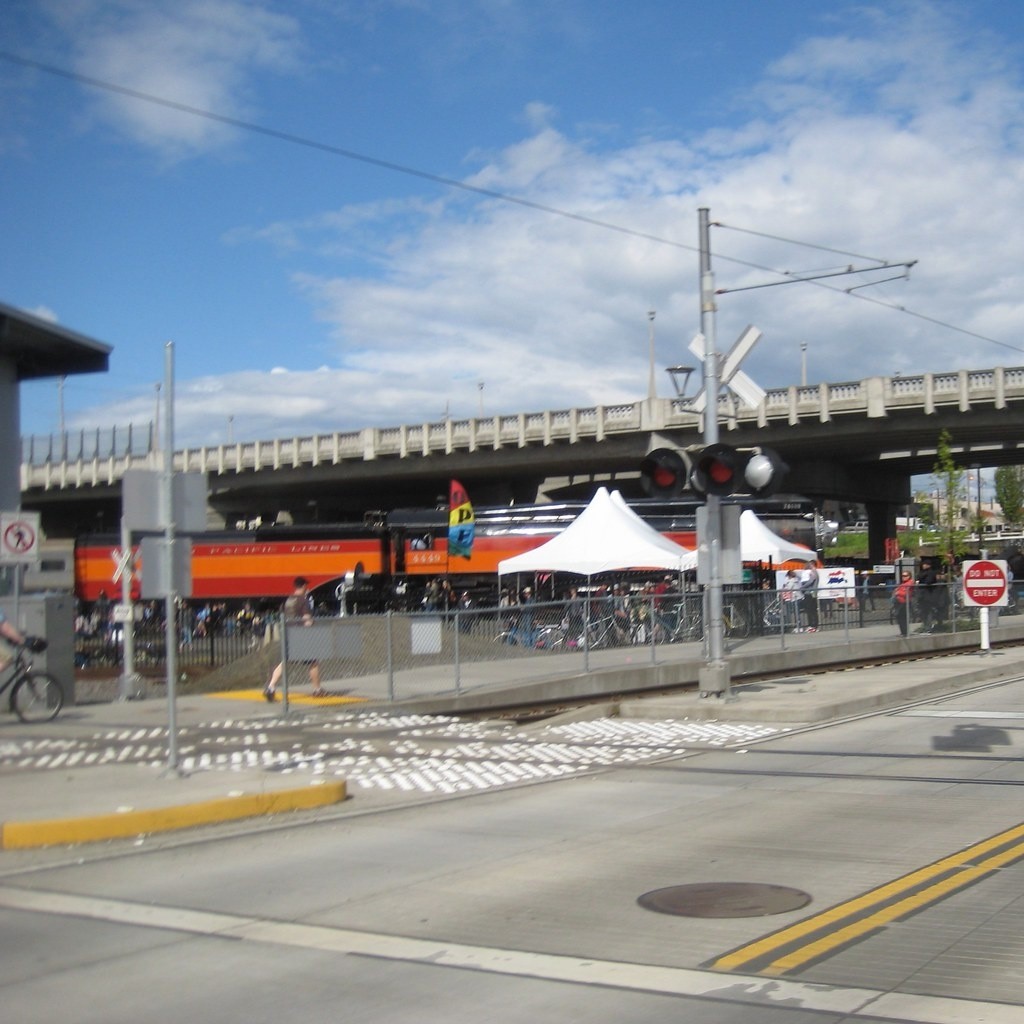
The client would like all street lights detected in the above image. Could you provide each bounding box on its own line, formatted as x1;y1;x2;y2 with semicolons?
800;341;808;386
648;304;655;400
478;382;484;418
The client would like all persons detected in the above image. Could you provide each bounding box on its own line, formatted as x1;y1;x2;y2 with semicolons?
860;571;876;612
70;597;267;670
780;560;834;636
421;578;480;633
0;610;47;674
262;576;334;702
891;559;1014;637
497;574;682;649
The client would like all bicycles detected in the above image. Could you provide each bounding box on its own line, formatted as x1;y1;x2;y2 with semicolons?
1;631;64;725
83;623;162;672
487;598;752;645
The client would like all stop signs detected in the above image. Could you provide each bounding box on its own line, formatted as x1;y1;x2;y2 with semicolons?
964;561;1007;604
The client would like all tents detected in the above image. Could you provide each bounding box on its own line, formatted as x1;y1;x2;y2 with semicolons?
680;510;819;631
499;488;692;633
610;490;693;557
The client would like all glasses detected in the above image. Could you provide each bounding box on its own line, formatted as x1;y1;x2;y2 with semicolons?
901;575;909;577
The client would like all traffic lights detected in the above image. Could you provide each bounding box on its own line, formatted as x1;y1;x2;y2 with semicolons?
639;449;687;499
695;443;746;496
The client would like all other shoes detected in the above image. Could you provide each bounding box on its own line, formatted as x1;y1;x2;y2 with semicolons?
804;626;819;633
791;628;803;633
897;634;907;638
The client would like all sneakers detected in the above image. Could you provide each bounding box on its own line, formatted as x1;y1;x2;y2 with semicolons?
314;687;333;697
263;688;275;702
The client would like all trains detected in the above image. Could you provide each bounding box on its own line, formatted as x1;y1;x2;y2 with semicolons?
73;493;838;621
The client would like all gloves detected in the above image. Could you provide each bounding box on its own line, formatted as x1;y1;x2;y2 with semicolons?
25;638;47;652
